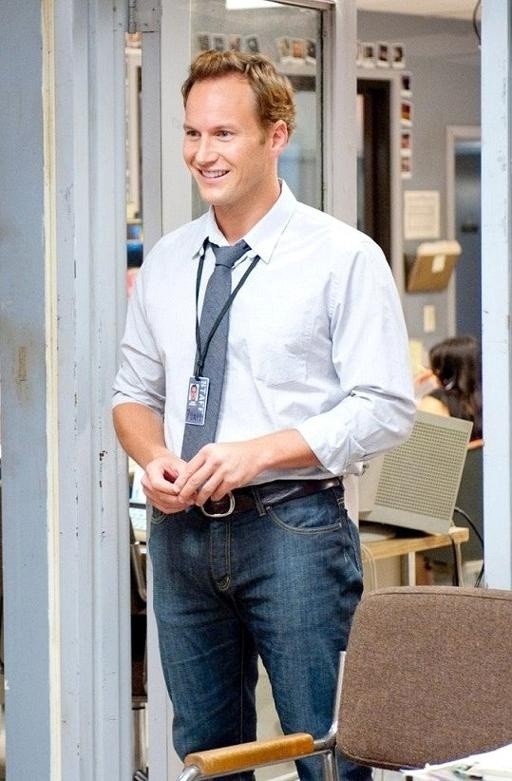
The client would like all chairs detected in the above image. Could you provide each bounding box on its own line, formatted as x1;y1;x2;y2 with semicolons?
179;582;512;780
0;456;150;781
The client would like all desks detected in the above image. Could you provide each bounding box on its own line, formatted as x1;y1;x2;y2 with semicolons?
352;514;476;588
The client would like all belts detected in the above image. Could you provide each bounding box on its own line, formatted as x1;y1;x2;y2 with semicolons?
198;477;342;520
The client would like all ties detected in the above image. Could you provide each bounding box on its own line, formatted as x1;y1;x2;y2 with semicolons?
180;239;251;462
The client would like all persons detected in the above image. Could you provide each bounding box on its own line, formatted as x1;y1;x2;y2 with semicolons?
414;335;483;444
112;47;418;780
